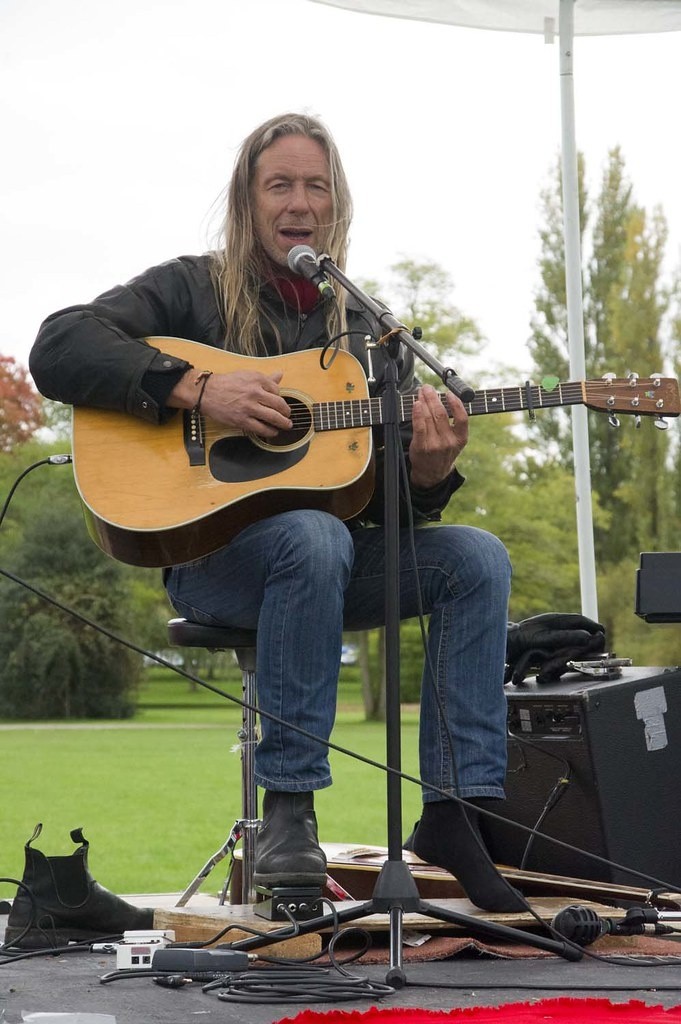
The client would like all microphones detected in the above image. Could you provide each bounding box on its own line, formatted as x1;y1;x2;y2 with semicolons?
551;904;676;946
287;244;336;301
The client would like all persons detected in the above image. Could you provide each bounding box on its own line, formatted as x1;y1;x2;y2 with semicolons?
27;113;534;915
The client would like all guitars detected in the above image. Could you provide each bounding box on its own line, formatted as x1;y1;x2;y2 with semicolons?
228;841;681;912
70;336;681;570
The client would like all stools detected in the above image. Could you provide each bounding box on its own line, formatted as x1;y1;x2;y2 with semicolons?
167;617;357;910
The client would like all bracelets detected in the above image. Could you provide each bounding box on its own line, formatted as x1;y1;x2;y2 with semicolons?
192;370;214;414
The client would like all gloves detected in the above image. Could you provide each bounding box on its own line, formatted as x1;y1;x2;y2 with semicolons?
508;611;605;685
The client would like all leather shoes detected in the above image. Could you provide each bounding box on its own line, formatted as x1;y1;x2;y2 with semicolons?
250;792;326;884
6;824;155;949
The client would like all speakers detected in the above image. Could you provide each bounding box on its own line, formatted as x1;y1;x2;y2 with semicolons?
503;666;681;909
